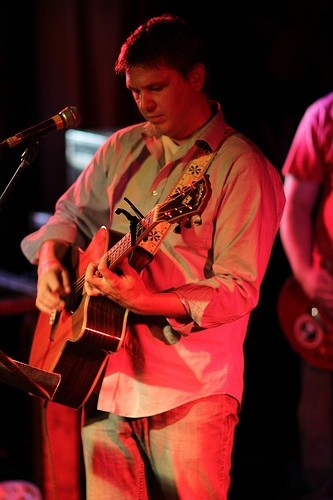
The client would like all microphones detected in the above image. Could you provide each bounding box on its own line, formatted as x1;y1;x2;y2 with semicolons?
0;105;81;151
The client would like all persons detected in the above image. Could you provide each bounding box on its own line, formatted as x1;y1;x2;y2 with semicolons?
278;92;333;500
19;15;286;500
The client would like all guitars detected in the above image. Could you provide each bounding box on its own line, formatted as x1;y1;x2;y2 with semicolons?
273;175;333;369
27;171;213;411
